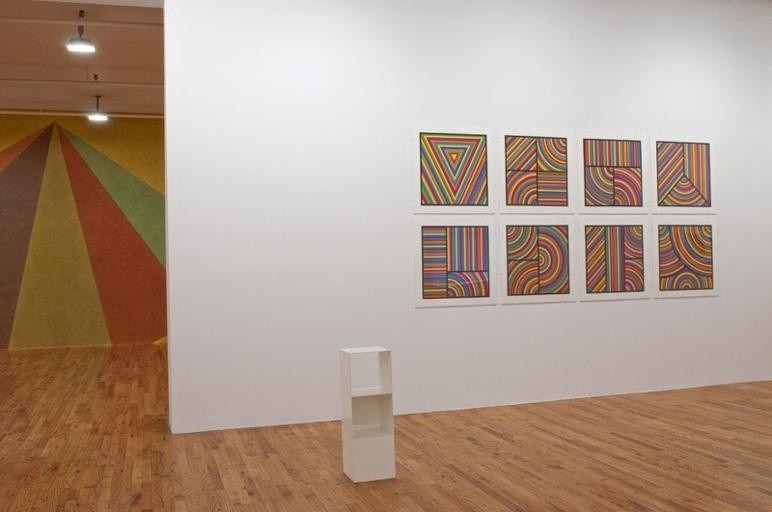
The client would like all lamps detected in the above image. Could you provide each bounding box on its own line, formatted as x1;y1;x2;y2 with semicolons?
65;10;108;123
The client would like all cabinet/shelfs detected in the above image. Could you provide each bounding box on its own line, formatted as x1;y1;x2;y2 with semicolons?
340;346;396;484
